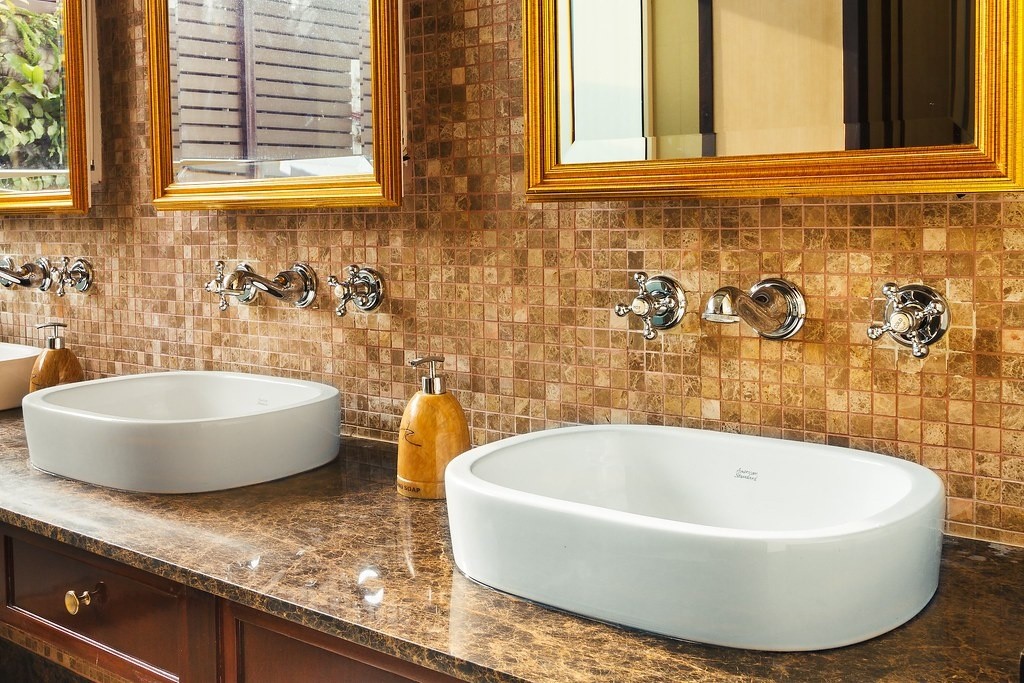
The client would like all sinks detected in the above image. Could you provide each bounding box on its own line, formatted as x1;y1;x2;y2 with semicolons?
444;423;946;652
0;342;46;411
20;369;341;495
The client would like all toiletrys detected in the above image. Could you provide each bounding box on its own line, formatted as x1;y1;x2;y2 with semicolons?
395;356;471;500
29;321;86;395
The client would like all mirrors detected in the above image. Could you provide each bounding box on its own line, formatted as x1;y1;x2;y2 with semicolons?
0;0;101;215
521;0;1024;199
145;0;407;211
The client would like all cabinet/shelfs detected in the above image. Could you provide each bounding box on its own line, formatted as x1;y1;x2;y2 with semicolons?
0;520;180;683
215;595;467;683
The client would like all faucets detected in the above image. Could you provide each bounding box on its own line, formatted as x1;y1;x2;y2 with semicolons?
222;264;317;309
0;257;51;292
698;277;808;341
